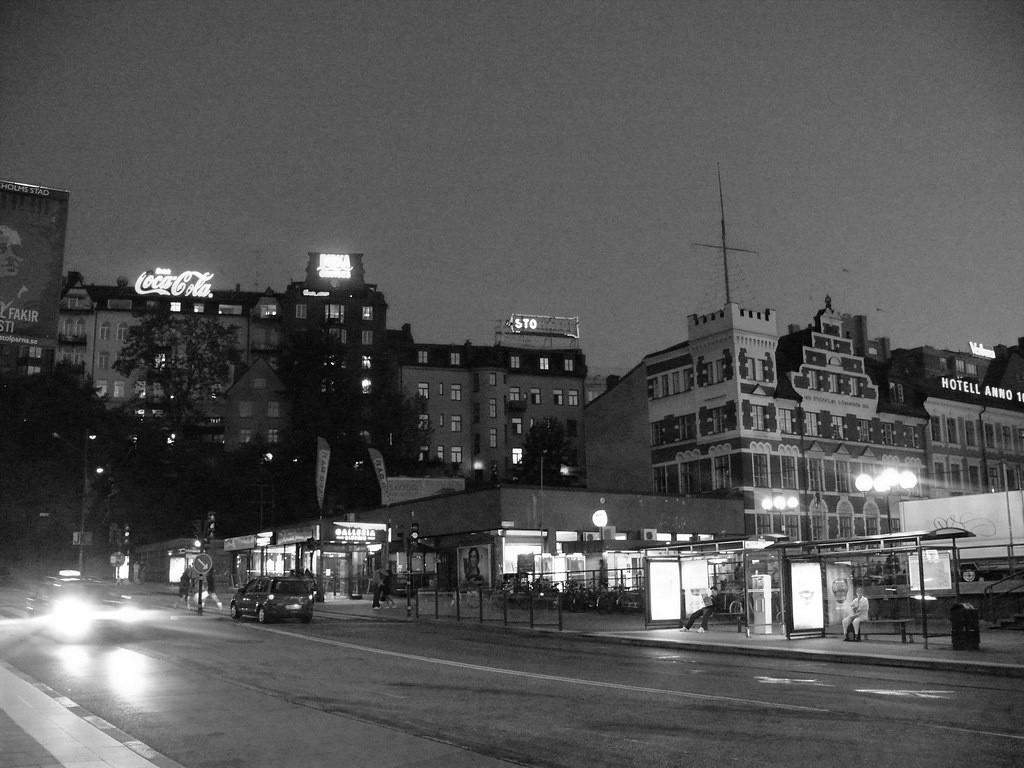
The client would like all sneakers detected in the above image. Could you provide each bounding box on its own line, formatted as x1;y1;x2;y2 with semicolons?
697;627;705;633
680;626;689;632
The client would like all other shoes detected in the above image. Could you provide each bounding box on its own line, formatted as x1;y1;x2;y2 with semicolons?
383;606;389;608
844;638;849;641
392;605;396;609
854;637;858;640
372;607;381;609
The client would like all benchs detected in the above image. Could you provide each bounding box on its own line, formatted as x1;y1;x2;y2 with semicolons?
857;618;916;643
703;612;746;633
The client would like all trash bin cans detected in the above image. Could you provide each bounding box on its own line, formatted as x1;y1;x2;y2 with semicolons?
949;602;980;651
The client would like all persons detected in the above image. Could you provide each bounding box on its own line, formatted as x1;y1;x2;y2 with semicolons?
372;565;397;611
842;587;870;642
174;569;190;610
288;570;316;604
598;560;609;590
679;586;723;633
201;566;222;611
465;547;481;584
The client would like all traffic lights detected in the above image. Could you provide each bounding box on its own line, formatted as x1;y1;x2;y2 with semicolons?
410;523;419;542
123;524;131;546
205;509;216;539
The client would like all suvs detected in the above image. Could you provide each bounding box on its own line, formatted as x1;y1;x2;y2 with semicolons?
228;572;315;622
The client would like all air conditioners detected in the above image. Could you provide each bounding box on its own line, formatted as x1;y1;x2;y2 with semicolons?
583;532;599;541
640;529;657;541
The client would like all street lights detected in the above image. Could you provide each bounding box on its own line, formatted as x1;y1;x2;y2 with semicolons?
540;449;547;579
854;465;918;627
761;495;799;535
77;429;97;574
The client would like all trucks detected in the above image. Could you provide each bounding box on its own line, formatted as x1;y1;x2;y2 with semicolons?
896;490;1024;584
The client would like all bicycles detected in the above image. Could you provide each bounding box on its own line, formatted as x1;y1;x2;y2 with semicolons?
489;572;787;625
